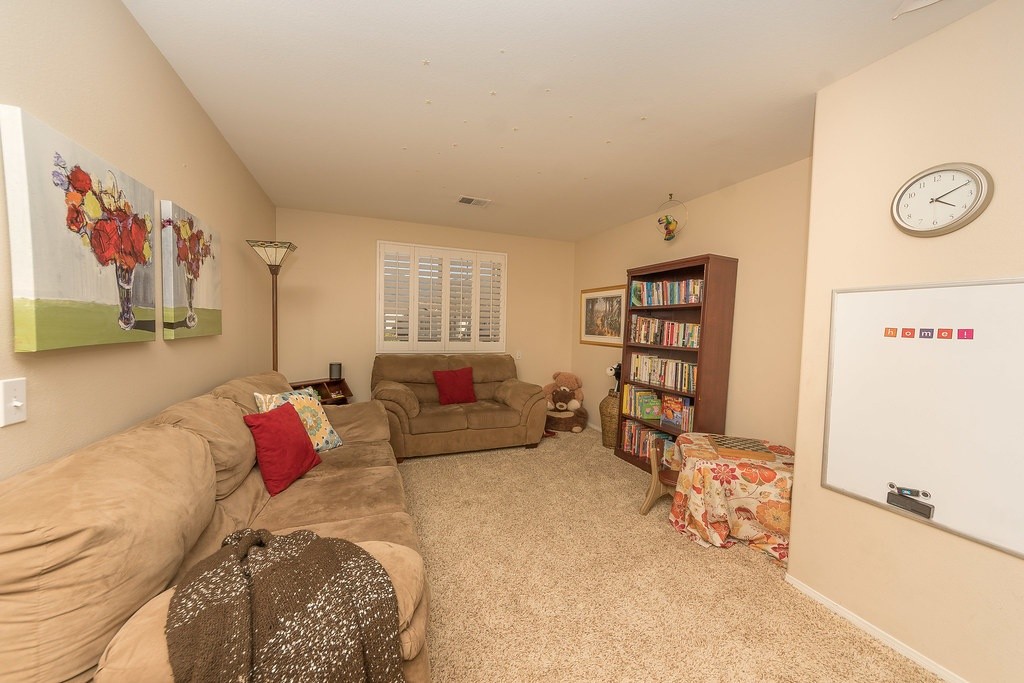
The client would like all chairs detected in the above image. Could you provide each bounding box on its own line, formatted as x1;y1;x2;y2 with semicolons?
639;437;679;515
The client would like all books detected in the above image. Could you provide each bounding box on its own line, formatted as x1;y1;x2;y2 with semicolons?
660;396;694;432
622;383;660;420
629;313;700;349
630;278;703;306
630;351;659;385
657;434;676;469
620;420;655;460
658;358;697;393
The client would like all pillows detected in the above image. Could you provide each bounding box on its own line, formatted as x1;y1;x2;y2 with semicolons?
433;367;477;405
243;402;322;497
255;386;343;452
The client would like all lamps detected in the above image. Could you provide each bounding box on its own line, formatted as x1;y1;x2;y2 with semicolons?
247;240;297;371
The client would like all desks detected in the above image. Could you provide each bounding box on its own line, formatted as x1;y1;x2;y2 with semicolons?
675;433;795;545
288;378;353;405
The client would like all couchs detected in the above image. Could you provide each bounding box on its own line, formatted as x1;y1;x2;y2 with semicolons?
371;354;547;462
0;372;431;683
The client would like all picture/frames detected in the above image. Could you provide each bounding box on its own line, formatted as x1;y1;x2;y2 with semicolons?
580;284;627;348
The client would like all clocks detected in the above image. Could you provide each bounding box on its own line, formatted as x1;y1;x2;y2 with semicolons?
891;162;994;238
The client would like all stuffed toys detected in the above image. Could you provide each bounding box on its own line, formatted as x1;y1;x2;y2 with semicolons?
543;371;589;433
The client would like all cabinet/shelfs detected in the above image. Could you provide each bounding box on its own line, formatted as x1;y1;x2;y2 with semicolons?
614;254;738;474
